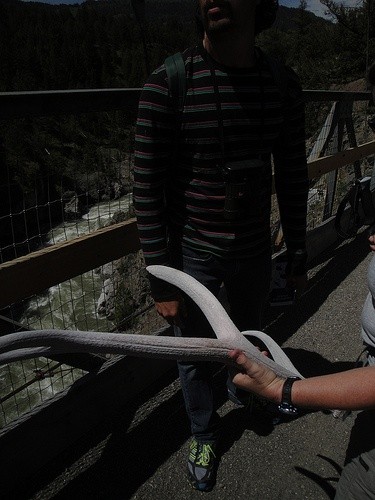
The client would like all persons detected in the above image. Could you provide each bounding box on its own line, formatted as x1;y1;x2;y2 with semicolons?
227;225;375;500
121;0;309;492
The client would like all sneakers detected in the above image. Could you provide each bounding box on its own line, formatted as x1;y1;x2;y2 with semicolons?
227;389;283;425
187;440;215;492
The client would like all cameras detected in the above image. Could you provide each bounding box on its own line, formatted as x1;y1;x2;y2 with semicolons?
222;159;264;219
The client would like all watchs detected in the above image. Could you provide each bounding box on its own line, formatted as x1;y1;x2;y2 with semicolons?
278;375;302;417
